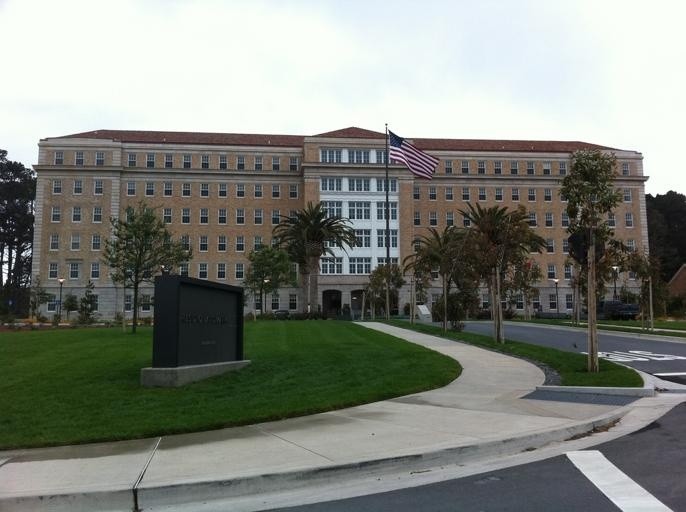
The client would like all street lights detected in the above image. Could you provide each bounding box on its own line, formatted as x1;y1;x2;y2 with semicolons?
58;276;65;323
264;277;271;313
555;278;560;313
612;266;618;299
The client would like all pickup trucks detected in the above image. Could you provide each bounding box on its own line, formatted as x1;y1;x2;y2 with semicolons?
597;299;639;321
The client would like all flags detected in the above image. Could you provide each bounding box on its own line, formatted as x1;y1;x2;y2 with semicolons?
388;130;440;181
